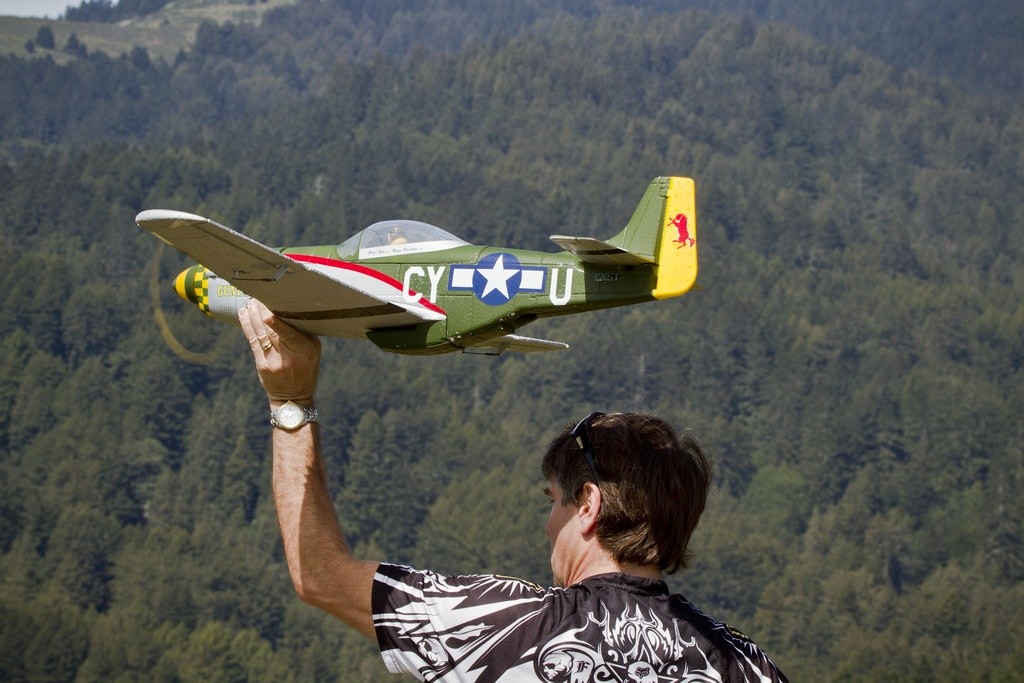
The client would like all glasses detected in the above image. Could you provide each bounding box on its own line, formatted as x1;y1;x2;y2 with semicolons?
571;412;601;492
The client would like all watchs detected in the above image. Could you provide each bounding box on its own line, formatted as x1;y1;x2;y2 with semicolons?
269;400;321;430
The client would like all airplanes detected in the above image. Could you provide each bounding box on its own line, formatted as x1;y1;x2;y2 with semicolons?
133;174;700;362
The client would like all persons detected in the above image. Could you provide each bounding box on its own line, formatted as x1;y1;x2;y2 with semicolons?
387;226;408;245
236;296;791;683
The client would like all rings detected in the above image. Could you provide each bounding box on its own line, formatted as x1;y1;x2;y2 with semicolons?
262;341;272;351
